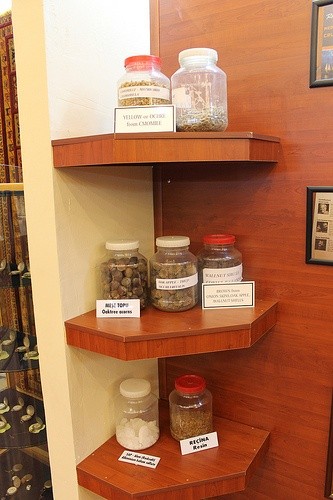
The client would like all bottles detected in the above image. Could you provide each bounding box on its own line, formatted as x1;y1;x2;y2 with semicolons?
148;236;198;312
171;47;229;132
196;233;244;302
99;239;149;310
116;55;171;105
115;377;160;451
169;373;213;443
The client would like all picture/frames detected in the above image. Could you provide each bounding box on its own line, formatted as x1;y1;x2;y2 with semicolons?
304;184;332;265
309;0;333;89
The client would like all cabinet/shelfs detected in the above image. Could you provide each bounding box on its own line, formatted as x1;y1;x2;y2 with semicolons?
0;12;51;500
50;129;282;498
